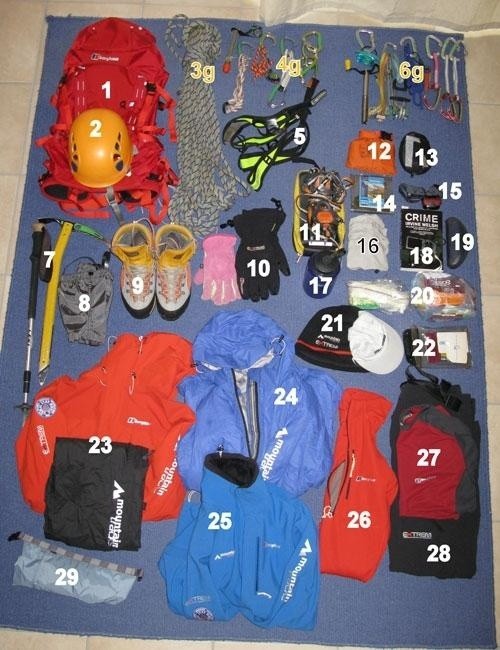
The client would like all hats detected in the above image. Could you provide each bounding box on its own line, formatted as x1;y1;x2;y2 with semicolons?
294;306;405;374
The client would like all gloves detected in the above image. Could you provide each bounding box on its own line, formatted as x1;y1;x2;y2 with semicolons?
193;198;291;306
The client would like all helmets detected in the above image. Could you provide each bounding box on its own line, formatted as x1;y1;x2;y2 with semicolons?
68;108;132;188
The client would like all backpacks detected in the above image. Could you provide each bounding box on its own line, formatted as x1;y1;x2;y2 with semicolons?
36;16;181;225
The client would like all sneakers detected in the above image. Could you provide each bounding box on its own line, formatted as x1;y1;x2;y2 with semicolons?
110;218;196;321
